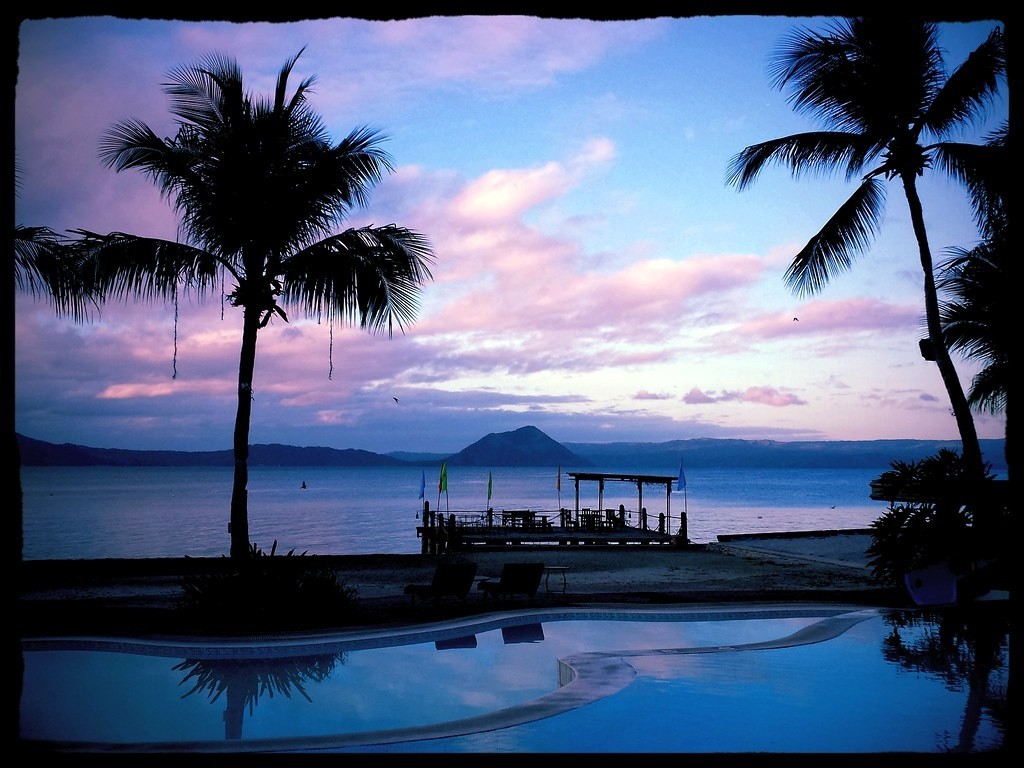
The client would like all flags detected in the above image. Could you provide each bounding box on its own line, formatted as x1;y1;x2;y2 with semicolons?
556;466;560;491
441;461;447;492
438;463;443;491
677;457;686;491
487;470;492;499
418;470;426;500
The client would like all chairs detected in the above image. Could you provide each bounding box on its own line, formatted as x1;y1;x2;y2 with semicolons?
502;507;617;533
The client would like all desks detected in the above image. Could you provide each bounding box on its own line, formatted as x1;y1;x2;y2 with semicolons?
502;513;537;529
578;514;605;530
543;566;569;594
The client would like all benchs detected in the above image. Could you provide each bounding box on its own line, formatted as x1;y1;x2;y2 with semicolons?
477;561;544;602
434;635;477;651
501;623;544;644
404;558;479;605
